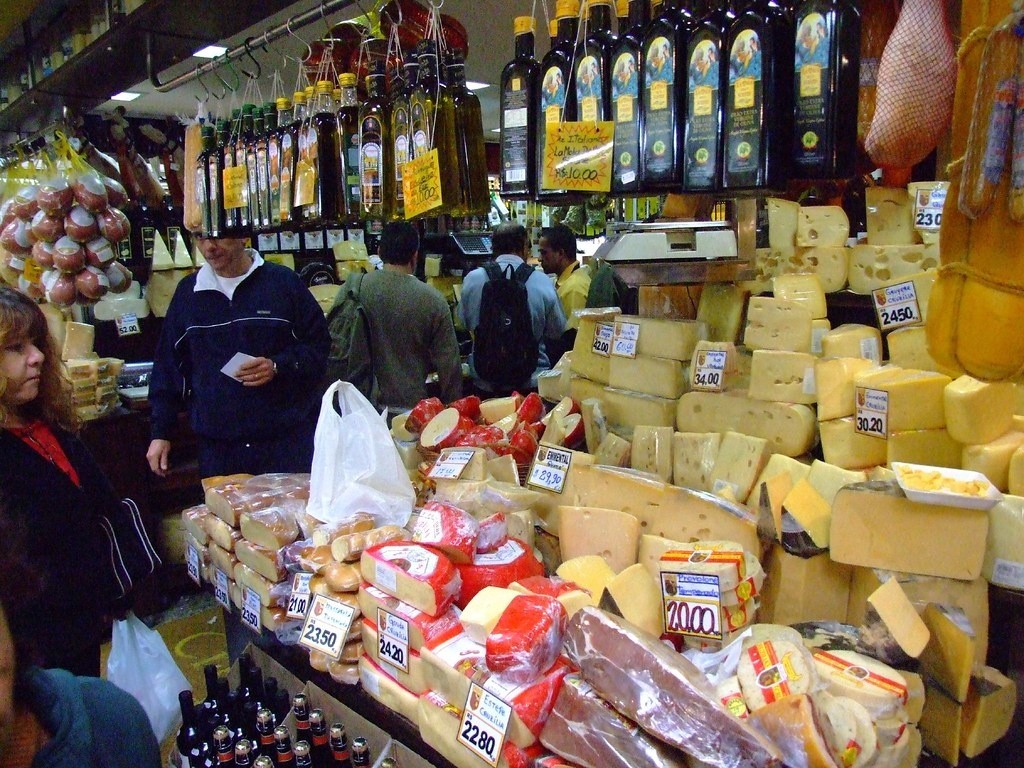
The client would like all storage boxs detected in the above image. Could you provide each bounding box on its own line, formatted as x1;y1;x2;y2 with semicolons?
252;680;392;768
166;641;306;768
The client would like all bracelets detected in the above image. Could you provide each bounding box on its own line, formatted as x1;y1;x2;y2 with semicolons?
273;361;279;375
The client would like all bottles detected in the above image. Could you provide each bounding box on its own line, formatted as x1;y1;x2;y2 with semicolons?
0;0;126;113
499;15;541;202
159;194;191;259
196;39;460;239
176;652;399;768
442;49;491;217
132;197;161;268
539;0;793;206
787;0;861;181
115;204;137;267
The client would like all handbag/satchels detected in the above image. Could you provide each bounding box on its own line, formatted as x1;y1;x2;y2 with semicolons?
105;609;193;746
326;273;369;381
305;378;415;527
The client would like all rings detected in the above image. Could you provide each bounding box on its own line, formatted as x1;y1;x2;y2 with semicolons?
255;374;259;380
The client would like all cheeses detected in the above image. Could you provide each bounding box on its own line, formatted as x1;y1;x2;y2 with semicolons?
180;178;1024;768
36;228;193;433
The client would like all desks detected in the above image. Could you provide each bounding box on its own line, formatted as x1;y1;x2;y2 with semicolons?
27;377;199;523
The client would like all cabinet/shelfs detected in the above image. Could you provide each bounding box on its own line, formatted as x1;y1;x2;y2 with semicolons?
1;0;302;154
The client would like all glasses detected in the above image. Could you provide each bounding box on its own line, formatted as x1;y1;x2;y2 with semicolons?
189;231;207;245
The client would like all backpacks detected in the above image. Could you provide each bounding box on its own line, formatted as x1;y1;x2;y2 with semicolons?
472;261;538;390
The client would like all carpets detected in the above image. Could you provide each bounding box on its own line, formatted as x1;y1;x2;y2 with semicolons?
99;606;230;768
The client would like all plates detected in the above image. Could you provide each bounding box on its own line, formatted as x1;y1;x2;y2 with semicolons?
891;461;1005;511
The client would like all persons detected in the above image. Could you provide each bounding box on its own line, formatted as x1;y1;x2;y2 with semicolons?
538;227;592;370
331;220;463;436
457;221;566;398
0;282;170;679
147;234;332;486
